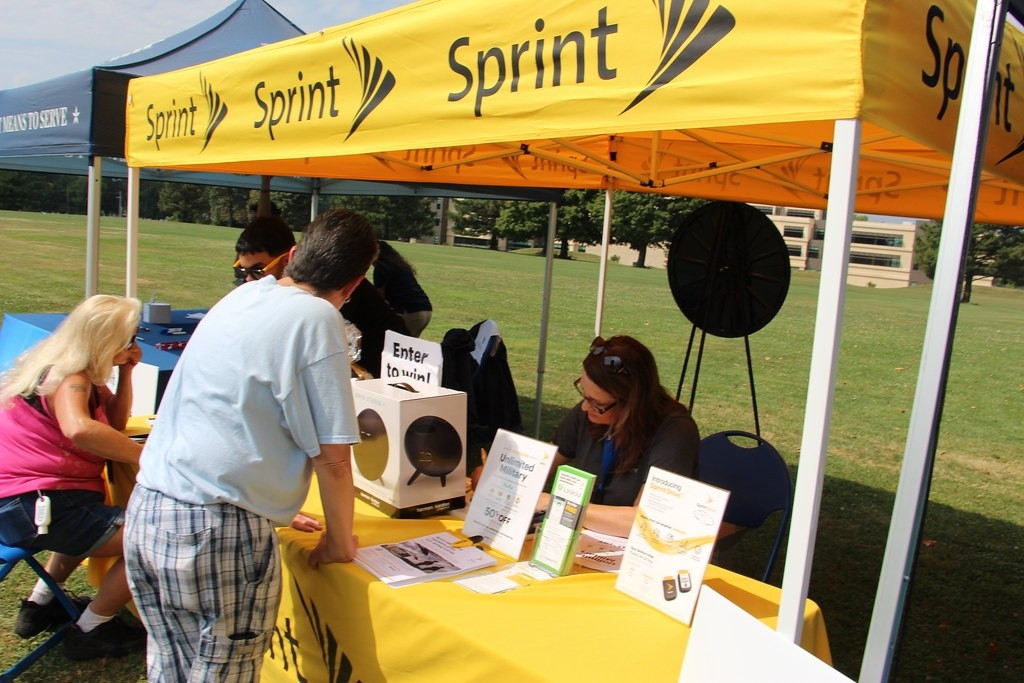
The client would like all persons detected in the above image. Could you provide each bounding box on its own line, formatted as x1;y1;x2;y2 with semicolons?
472;335;699;539
372;239;432;338
232;200;408;377
123;208;373;683
1;294;146;660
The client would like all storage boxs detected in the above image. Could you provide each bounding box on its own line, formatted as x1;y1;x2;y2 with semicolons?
349;378;468;522
143;302;172;324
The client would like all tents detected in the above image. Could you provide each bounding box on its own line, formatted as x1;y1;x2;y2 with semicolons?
0;0;565;438
124;0;1023;683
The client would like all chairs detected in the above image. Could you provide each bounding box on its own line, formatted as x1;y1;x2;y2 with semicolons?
697;428;795;583
442;318;522;446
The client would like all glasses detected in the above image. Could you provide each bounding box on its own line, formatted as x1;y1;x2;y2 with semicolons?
232;253;289;281
574;378;619;415
590;336;634;381
123;335;136;351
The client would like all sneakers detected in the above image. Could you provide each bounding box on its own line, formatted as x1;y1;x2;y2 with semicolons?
62;614;147;661
13;589;91;639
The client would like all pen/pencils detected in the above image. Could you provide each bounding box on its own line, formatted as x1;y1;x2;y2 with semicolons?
480;447;488;466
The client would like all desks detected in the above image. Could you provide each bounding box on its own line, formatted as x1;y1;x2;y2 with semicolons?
101;410;835;683
0;308;215;413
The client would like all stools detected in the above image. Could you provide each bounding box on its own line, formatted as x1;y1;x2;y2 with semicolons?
0;544;85;683
